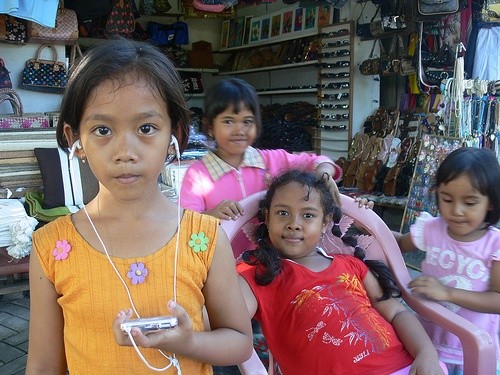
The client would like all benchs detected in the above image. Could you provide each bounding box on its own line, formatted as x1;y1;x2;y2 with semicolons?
0;150;53;295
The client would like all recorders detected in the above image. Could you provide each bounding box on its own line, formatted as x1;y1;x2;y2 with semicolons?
118;316;177;333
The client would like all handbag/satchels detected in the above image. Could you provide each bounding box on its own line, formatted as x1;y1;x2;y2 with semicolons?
146;20;190;46
0;13;25;45
417;0;460;16
398;56;416;77
370;20;384;36
177;71;204;95
414;44;455;69
355;22;372;38
382;16;407;30
139;0;172;17
25;0;79;45
0;88;50;130
89;0;136;37
20;43;69;94
358;55;389;76
416;69;458;95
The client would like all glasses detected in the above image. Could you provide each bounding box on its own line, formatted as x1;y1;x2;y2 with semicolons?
318;28;350;101
332;124;347;131
315;124;331;131
321;104;333;110
320;114;331;120
333;104;349;109
335;113;349;120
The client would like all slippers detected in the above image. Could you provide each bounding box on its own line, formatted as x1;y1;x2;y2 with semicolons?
332;107;416;197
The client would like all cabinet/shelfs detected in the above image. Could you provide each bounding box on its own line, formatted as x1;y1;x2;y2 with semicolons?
64;7;340;152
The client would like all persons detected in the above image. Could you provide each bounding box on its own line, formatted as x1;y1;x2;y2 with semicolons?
352;146;500;375
26;40;254;375
177;78;343;264
202;168;446;375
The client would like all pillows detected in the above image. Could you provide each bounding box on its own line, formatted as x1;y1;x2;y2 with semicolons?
34;147;99;209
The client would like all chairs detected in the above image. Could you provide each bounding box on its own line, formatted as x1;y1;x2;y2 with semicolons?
218;189;497;375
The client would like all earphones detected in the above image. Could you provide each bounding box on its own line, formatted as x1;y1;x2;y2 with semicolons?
75;140;82;149
169;135;177;145
